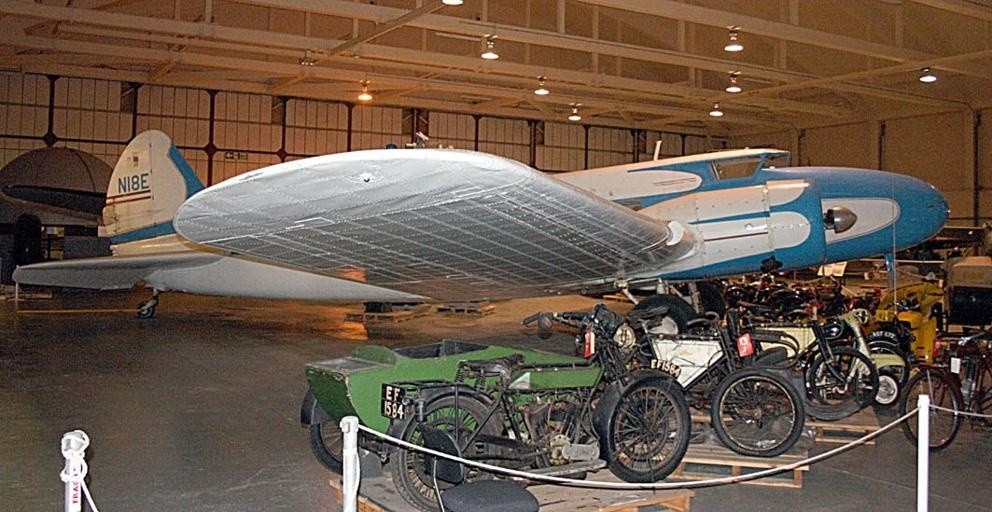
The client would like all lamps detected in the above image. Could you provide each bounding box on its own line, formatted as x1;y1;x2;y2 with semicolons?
920;66;938;83
479;32;583;122
709;23;745;118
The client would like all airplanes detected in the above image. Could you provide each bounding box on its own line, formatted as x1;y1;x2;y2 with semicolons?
11;130;948;327
